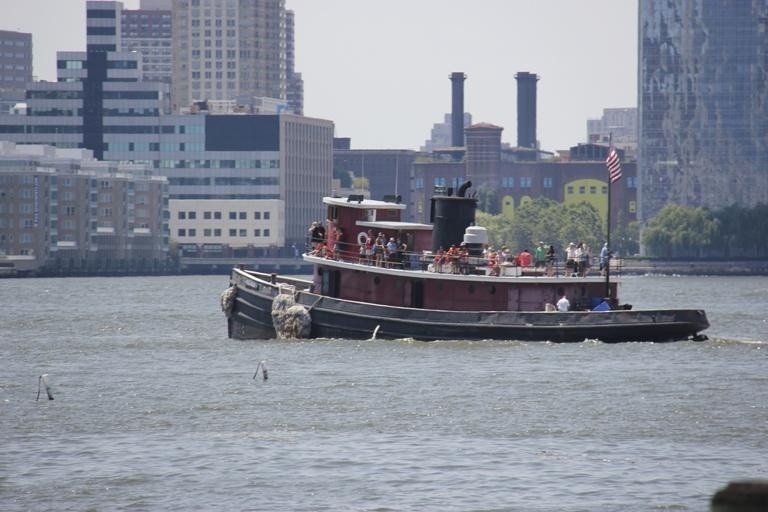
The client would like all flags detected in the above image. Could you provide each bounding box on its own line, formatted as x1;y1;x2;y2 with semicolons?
606;145;622;183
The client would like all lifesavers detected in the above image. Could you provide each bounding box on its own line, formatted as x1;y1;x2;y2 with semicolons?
357;232;369;246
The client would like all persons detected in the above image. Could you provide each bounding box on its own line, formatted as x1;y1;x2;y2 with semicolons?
481;239;555;278
556;295;571;312
433;245;471;276
564;242;611;277
306;218;343;263
358;230;408;270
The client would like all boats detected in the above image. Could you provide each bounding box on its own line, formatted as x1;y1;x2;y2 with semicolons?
221;179;710;344
